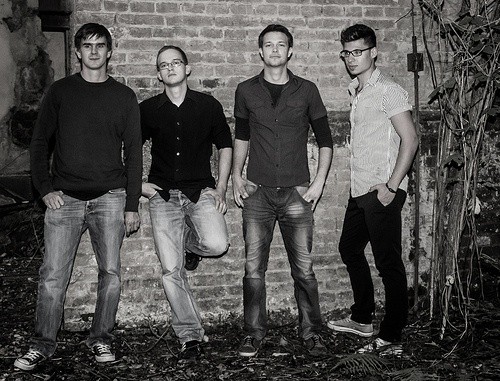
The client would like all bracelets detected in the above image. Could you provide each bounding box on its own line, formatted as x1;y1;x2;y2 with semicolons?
385;183;396;194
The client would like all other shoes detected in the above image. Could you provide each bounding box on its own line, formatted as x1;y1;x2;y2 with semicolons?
184;250;202;270
181;340;201;359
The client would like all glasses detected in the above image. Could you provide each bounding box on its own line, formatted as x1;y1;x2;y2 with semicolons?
158;60;186;69
340;47;373;58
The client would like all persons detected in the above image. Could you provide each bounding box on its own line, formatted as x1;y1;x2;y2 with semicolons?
139;45;234;358
14;24;142;372
325;24;419;359
233;24;334;356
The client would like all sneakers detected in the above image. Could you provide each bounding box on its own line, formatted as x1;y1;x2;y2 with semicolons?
355;338;402;355
91;344;115;363
327;314;373;337
303;332;327;356
14;348;45;370
239;335;262;356
